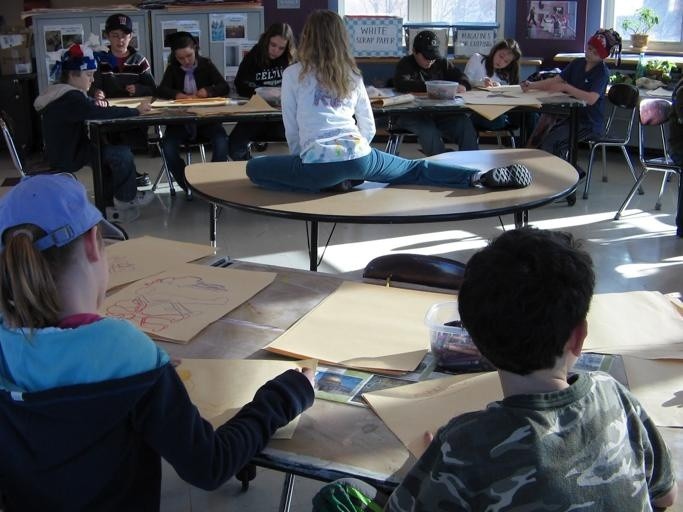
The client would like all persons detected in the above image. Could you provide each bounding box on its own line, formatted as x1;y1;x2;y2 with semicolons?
391;27;620;182
245;10;531;191
30;9;297;224
313;224;676;512
0;171;316;510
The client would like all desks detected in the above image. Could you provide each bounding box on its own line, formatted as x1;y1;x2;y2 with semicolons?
95;234;683;511
183;146;580;270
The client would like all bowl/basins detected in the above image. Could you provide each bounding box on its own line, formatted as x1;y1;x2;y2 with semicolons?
424;80;459;100
419;297;492;374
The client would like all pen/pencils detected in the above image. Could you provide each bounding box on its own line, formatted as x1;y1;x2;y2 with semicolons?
210;256;230;267
430;321;486;371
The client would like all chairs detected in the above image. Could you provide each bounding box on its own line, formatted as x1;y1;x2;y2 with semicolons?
279;253;469;511
577;85;644;199
88;77;585;215
3;121;81;184
616;97;680;221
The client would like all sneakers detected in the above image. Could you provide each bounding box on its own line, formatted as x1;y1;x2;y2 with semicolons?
481;164;531;188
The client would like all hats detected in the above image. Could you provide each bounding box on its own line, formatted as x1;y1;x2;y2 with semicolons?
415;31;441;59
106;15;132;34
1;174;127;252
53;45;96;77
590;29;620;58
